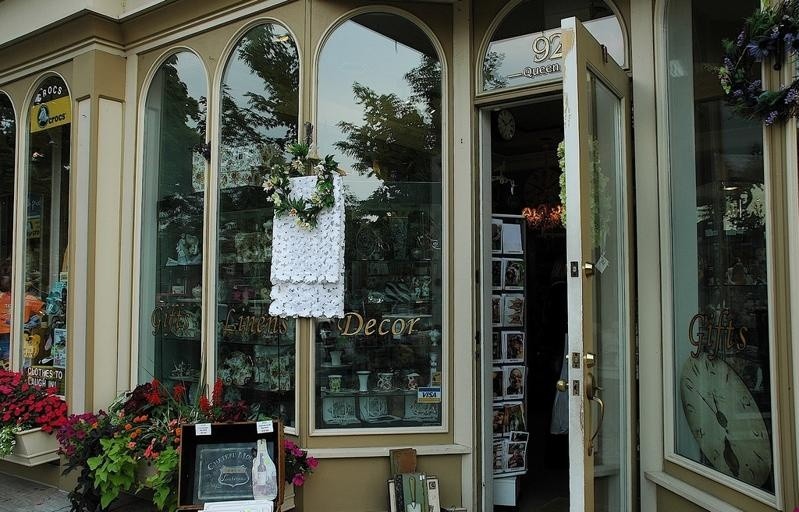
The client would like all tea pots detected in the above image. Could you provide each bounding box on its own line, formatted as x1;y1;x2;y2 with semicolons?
728;261;749;287
172;360;191;377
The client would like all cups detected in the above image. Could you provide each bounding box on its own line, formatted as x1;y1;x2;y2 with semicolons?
354;370;371;394
406;374;419;390
330;350;344;365
328;375;341;391
371;372;393;390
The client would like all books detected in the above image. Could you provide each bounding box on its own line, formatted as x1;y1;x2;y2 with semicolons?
491;215;533;480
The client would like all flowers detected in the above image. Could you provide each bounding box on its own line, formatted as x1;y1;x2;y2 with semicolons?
1;371;67;463
56;376;319;485
711;1;797;127
263;137;342;229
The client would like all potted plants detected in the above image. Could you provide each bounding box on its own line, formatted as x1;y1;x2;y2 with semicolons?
0;427;60;467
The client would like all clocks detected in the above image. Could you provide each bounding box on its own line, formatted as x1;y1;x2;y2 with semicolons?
678;348;773;493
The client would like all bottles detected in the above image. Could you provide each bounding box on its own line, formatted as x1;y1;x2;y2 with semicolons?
705;263;713;287
258;450;267;492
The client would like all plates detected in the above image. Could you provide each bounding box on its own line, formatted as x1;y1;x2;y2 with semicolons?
325;388;355;395
320;362;351;368
223;351;254;385
371;387;400;393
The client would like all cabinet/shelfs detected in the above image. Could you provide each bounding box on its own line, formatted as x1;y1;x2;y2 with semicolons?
155;186;442;430
